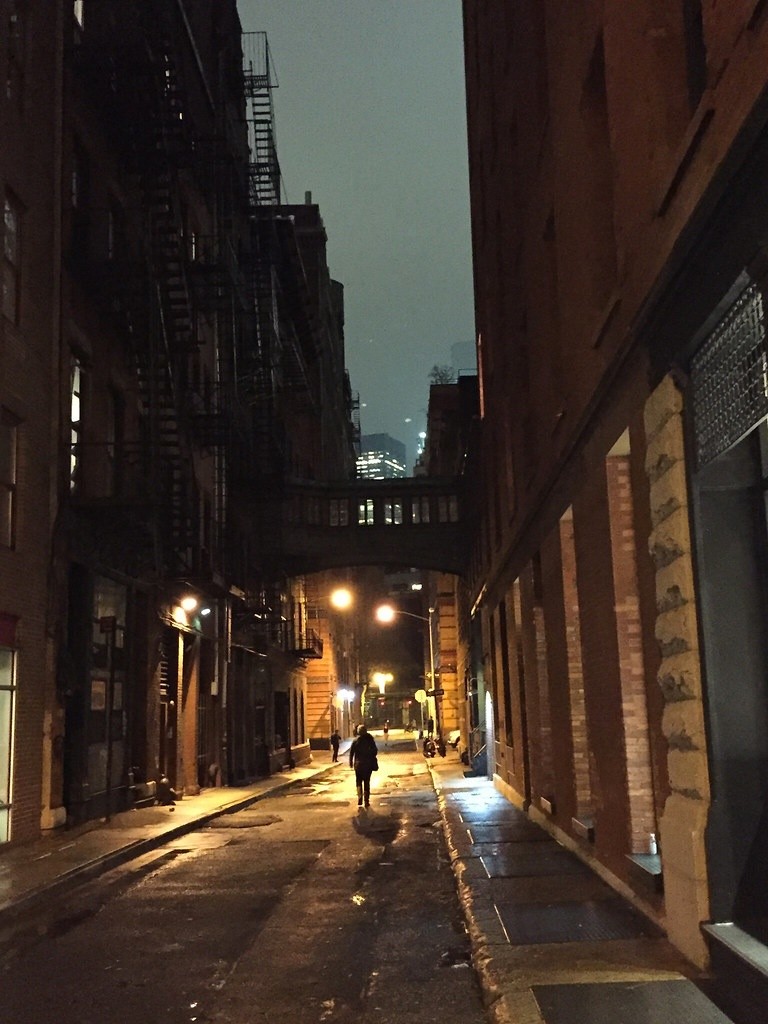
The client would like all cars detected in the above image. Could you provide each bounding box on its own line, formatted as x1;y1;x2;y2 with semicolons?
448;730;460;748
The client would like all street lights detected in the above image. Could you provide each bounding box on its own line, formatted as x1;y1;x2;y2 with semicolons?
380;607;440;736
305;594;346;616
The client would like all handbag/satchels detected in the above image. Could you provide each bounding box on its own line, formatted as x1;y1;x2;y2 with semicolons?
372;757;378;771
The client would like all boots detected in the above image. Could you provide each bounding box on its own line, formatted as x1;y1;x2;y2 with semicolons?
357;787;362;805
364;791;370;807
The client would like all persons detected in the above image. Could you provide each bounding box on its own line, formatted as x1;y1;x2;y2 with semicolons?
428;715;434;740
349;725;378;808
384;719;389;746
331;730;341;762
404;718;418;734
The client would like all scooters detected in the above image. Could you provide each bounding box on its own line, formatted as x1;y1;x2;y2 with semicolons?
433;737;447;758
422;736;436;758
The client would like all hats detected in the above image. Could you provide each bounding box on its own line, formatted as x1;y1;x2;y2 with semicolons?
357;725;366;734
